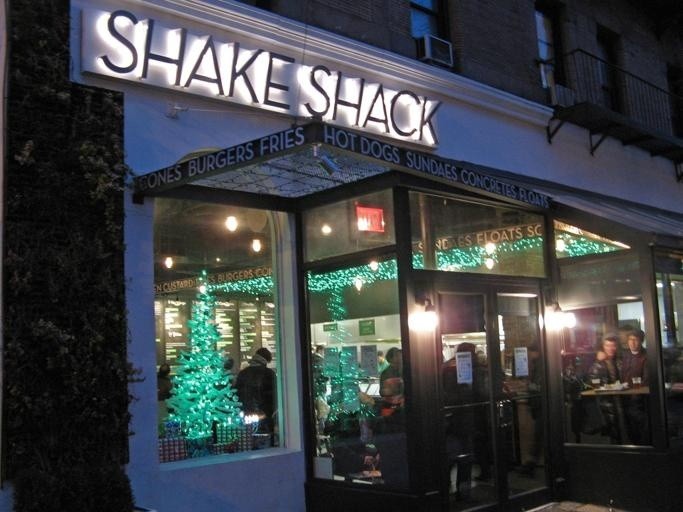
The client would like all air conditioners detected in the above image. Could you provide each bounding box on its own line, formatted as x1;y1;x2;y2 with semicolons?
413;34;454;69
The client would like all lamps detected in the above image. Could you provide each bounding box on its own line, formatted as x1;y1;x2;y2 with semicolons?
542;289;576;334
408;280;441;337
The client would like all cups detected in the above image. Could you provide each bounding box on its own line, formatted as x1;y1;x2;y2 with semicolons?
631;376;642;390
591;378;600;389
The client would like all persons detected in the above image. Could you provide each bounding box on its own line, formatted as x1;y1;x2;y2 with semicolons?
596;329;647;445
443;342;476;507
311;345;328;397
157;364;172;399
587;332;625;446
376;350;388;376
361;377;405;417
379;347;402;398
475;349;487;418
520;345;544;477
222;357;234;371
619;324;633;360
237;347;276;430
